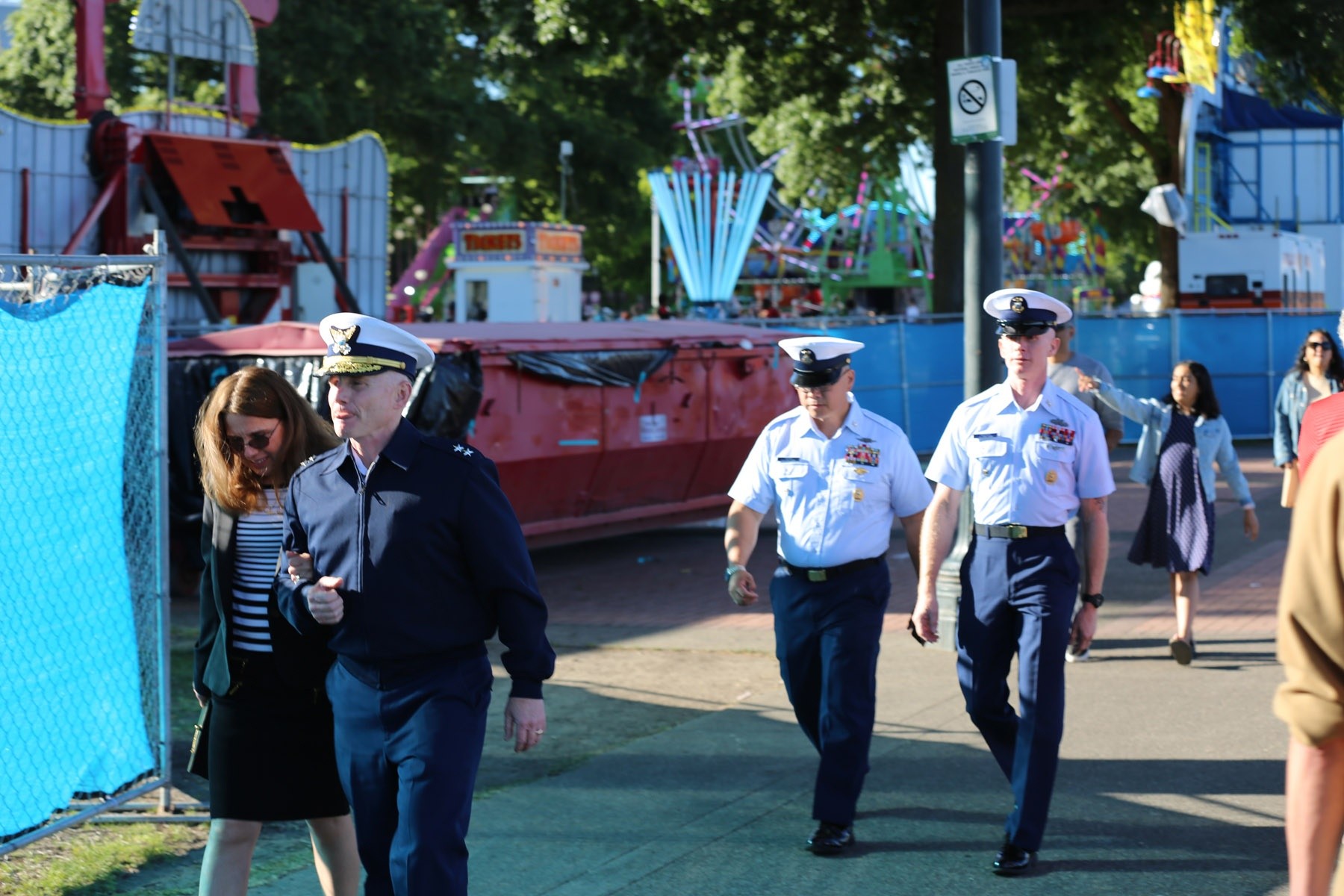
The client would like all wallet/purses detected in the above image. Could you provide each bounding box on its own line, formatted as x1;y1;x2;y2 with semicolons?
187;700;210;779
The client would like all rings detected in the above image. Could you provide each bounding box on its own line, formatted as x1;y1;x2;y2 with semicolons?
535;730;544;734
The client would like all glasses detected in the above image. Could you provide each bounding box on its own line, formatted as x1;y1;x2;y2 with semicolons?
1309;341;1332;351
1056;324;1072;332
225;418;281;452
788;368;851;392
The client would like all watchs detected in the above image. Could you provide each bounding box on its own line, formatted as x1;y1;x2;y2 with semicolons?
1083;593;1105;609
724;565;746;584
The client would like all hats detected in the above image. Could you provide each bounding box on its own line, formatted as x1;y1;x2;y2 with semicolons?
777;336;865;386
982;288;1072;336
311;311;436;380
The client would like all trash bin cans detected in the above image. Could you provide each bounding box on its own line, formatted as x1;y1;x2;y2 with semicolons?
159;316;818;557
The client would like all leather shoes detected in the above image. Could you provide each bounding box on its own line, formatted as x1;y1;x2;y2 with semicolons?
807;822;855;851
992;839;1037;870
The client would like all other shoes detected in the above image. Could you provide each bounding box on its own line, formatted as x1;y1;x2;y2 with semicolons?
1169;638;1193;666
1066;642;1089;663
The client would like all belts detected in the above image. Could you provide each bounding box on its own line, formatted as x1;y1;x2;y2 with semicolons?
971;523;1067;539
778;551;887;583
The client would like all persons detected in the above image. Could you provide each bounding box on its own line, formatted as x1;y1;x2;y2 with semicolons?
727;337;935;858
273;312;556;896
1044;313;1125;663
908;287;1119;879
1274;308;1344;895
1073;361;1260;666
188;366;351;896
